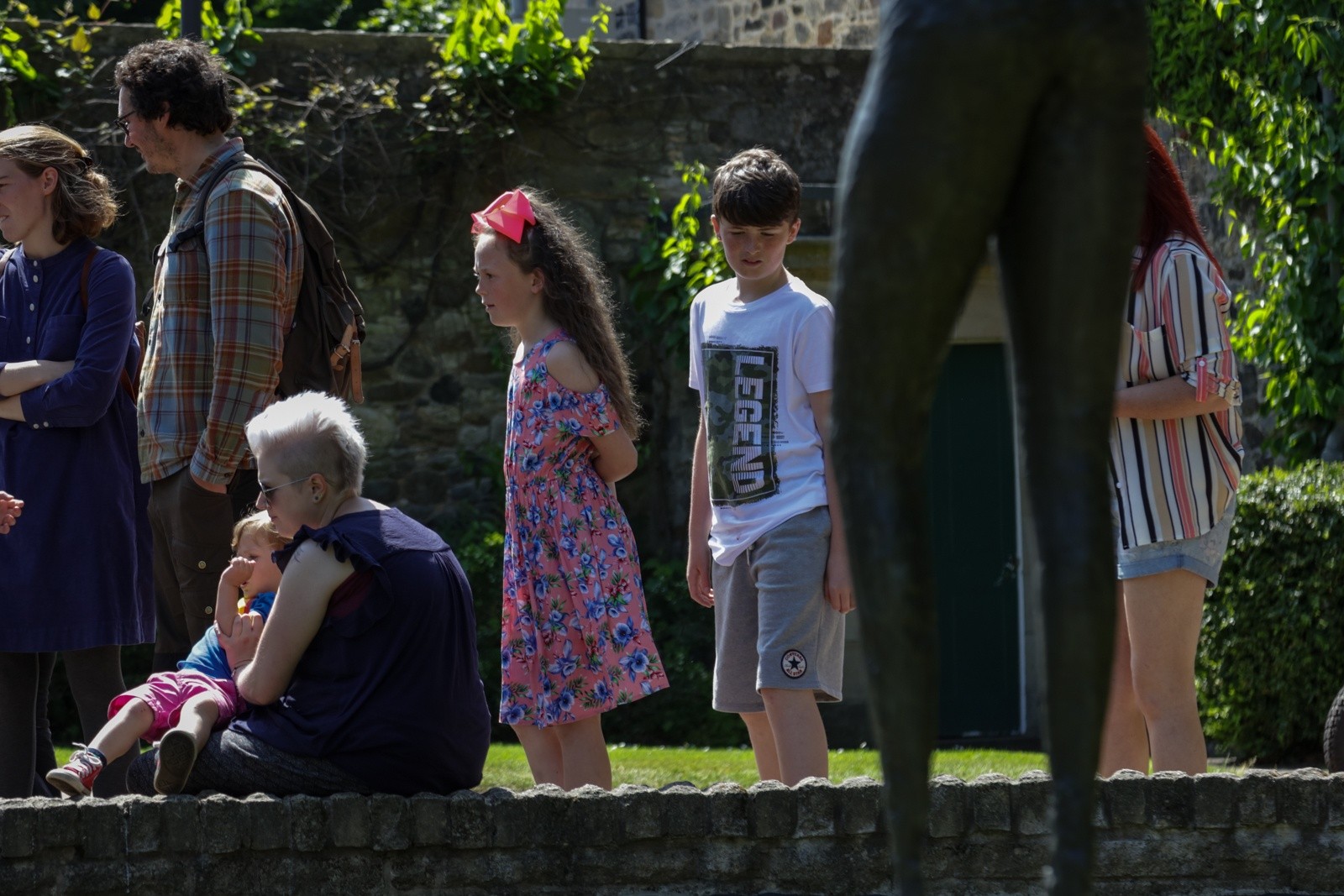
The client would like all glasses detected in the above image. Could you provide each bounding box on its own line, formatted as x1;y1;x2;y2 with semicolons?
258;475;332;503
114;110;138;136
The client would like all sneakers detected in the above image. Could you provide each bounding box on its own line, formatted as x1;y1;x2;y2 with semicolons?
46;742;104;797
153;727;198;796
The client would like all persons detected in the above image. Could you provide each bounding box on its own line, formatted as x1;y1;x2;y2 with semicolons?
128;393;489;794
0;126;154;805
686;150;856;785
117;39;303;676
471;186;671;792
46;510;293;794
1094;127;1242;778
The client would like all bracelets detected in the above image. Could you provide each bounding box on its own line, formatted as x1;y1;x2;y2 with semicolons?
229;663;252;677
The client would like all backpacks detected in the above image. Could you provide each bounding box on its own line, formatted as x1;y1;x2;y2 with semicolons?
0;246;147;407
192;157;366;406
